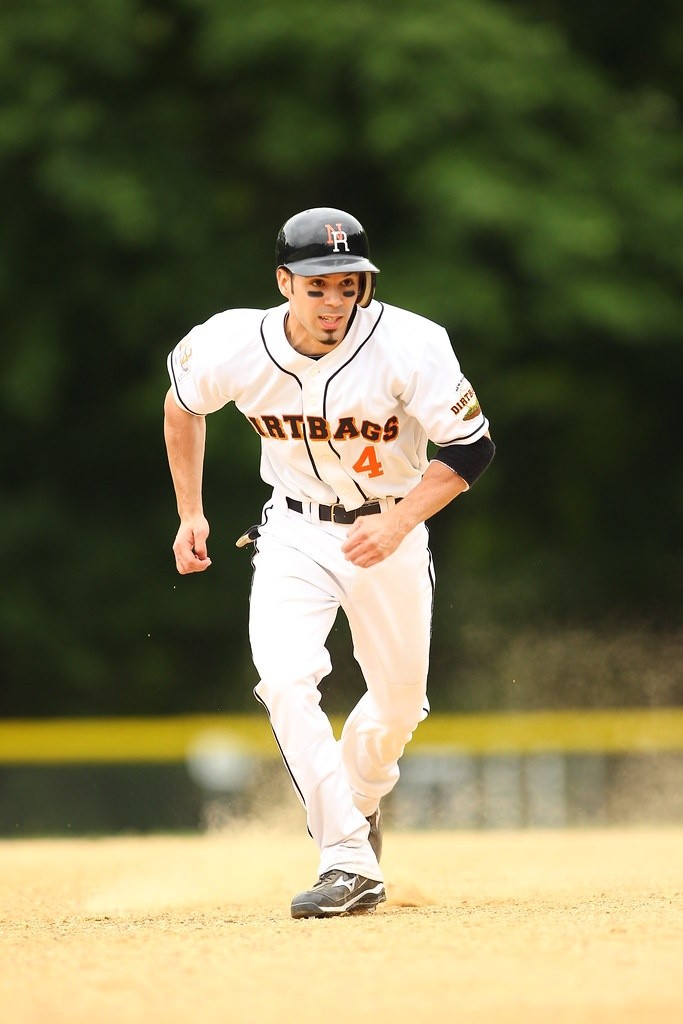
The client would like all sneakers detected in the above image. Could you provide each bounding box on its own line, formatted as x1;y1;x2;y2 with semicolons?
366;806;382;864
290;872;387;917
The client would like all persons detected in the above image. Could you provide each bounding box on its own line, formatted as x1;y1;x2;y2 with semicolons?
164;207;497;918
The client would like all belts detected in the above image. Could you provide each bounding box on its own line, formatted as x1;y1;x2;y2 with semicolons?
286;497;404;526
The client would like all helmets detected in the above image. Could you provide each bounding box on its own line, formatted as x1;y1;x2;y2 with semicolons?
274;207;379;308
